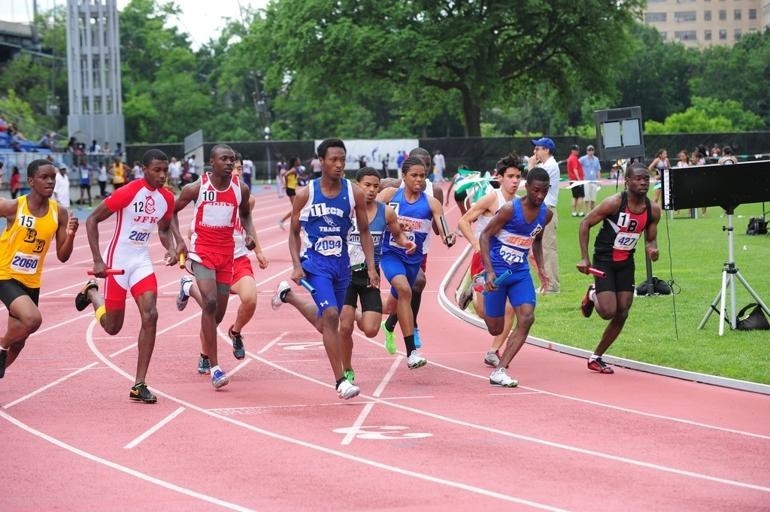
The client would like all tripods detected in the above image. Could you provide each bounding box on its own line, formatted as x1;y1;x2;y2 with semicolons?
698;214;770;335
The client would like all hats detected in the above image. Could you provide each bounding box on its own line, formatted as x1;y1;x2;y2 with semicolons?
530;138;557;153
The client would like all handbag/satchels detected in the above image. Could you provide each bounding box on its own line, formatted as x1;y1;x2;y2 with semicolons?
746;219;767;234
737;305;770;331
637;277;672;296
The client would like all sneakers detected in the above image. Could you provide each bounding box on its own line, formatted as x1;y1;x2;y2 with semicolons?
382;319;427;369
0;346;9;378
176;276;192;311
75;280;99;311
459;284;473;311
485;349;519;388
588;356;614;374
581;285;594;318
130;382;157;403
198;354;229;388
272;281;290;310
335;368;360;400
228;325;245;359
